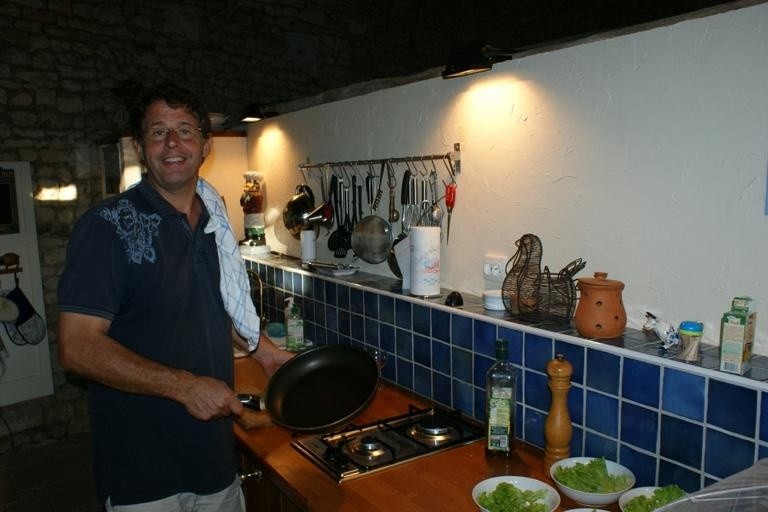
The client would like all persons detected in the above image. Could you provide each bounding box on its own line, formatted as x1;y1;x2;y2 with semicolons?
55;83;295;511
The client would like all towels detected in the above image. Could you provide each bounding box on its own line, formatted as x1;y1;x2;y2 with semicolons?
196;176;261;352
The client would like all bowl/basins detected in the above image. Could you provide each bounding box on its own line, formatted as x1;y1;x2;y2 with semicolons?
549;456;635;507
472;476;560;512
564;508;611;512
619;487;689;512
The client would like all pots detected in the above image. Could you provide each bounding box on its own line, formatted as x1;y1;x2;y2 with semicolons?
283;184;312;240
234;343;379;435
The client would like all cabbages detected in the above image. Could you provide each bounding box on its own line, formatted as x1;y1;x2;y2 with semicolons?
554;455;631;494
622;483;685;512
477;481;549;512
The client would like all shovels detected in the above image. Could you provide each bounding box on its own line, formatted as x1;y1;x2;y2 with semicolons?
333;178;346;258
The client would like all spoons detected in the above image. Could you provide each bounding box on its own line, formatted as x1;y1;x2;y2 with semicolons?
428;175;442;224
390;210;399;222
327;176;348;252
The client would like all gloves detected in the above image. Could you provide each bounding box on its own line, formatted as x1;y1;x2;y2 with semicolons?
0;293;28;345
5;285;46;345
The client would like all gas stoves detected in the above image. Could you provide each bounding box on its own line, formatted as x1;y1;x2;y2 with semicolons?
290;403;487;484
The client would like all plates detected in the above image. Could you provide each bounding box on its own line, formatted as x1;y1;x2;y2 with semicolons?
315;266;357;276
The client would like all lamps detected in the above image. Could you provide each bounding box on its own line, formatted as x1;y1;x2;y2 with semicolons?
442;27;492;80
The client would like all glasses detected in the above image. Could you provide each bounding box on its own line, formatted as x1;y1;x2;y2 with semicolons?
143;124;201;139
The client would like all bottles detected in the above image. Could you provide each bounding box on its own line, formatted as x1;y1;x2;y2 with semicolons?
299;221;317;262
484;339;517;458
285;307;304;348
